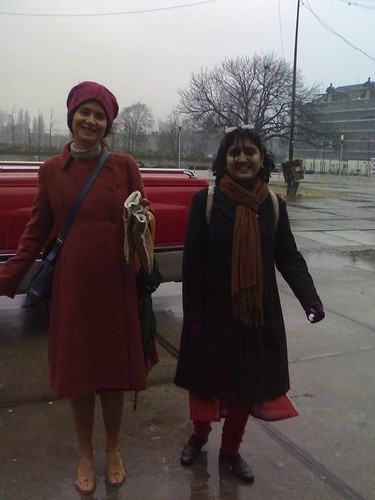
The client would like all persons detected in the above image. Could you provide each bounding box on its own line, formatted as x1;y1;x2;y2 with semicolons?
172;125;325;482
0;81;154;493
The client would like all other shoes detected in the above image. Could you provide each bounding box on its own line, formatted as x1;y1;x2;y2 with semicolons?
181;433;208;466
219;448;254;482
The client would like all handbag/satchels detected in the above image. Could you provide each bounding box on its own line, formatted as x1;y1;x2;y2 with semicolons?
27;239;63;306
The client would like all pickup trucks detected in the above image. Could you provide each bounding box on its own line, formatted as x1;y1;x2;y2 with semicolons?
0;160;211;294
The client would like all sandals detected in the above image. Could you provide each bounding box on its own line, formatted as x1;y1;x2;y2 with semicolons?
105;448;126;488
76;451;97;494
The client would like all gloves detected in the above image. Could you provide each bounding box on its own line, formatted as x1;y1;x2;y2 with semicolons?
304;300;325;324
123;191;154;276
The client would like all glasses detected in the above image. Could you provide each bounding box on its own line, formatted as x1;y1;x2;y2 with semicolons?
224;124;255;134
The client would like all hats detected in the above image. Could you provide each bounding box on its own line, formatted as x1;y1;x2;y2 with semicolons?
67;81;119;130
